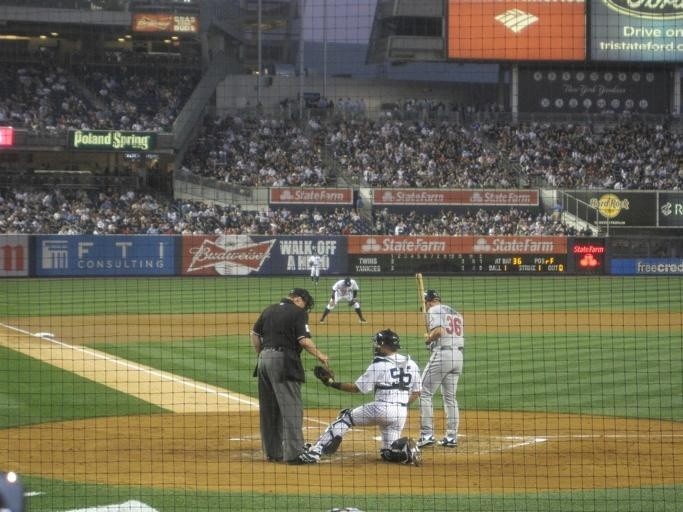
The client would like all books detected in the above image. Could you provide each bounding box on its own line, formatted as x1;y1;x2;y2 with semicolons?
182;96;683;191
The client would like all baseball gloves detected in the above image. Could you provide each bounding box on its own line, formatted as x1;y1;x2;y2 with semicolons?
314;366;334;386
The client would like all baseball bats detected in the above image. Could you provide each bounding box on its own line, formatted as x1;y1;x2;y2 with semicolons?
414;272;430;345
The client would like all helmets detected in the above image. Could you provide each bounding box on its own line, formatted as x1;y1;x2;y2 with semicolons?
291;287;313;306
375;329;400;349
419;289;441;311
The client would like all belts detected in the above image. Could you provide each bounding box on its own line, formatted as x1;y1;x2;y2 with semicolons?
440;344;464;352
261;346;293;352
382;400;408;407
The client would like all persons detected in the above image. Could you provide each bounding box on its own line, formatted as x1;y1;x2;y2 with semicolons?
298;328;424;466
308;249;322;284
415;290;465;448
319;278;369;324
0;162;592;238
249;287;331;466
0;46;202;135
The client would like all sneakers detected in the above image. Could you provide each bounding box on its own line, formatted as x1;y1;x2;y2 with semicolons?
265;445;323;464
404;438;421;466
416;434;435;447
438;436;457;447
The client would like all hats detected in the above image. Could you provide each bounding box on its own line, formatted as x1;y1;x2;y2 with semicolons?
344;277;351;286
